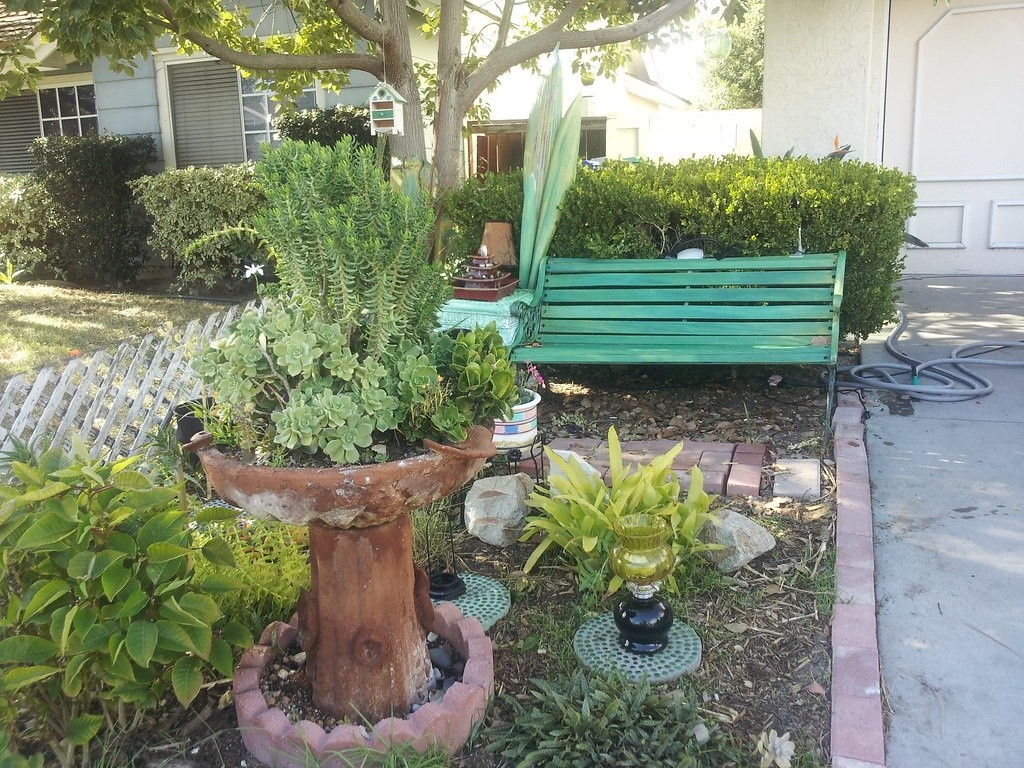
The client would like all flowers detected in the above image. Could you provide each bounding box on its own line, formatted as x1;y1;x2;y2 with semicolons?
517;361;545;402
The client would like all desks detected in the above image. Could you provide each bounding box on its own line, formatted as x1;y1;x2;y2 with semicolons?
435;287;536;348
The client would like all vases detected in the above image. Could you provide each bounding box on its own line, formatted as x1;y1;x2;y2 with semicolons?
491;389;542;453
172;396;215;465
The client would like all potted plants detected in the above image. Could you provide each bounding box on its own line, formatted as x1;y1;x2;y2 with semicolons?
178;129;517;720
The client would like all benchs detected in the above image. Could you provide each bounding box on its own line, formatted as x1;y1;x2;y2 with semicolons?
509;247;847;449
